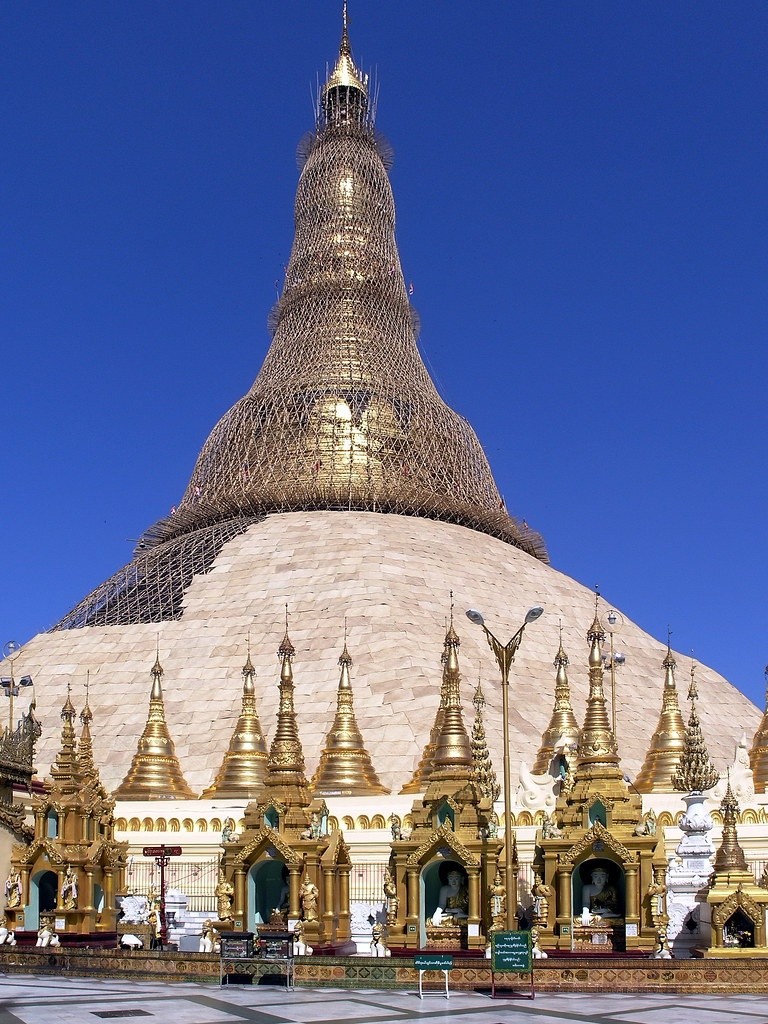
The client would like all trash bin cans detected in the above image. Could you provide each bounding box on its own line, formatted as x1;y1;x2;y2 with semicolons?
179;934;202;952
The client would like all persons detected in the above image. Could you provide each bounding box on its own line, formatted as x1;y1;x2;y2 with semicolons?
222;821;232;842
487;872;506;926
648;870;668;922
531;874;551;929
384;877;399;925
582;865;618;925
5;869;22;907
391;814;400;840
433;870;469;926
275;877;291;913
61;867;79;908
215;873;235;922
299;875;319;920
645;810;656;834
543;815;561;836
488;812;498;836
310;815;321;840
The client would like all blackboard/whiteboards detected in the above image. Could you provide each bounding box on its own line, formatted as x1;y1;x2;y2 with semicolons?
491;930;533;974
